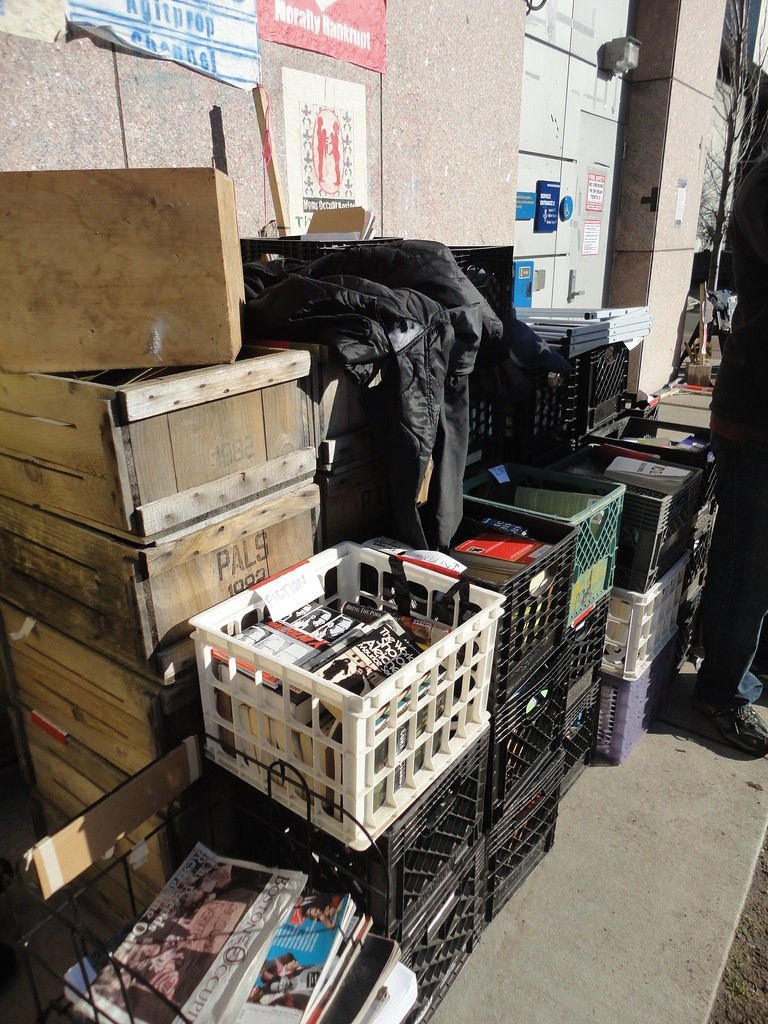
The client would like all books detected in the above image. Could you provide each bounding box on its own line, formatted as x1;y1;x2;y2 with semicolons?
638;435;715;460
512;486;605;537
604;455;693;494
601;443;660;459
59;843;418;1024
219;602;456;811
449;533;556;641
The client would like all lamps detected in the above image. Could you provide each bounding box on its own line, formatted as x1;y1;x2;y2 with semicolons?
604;36;642;76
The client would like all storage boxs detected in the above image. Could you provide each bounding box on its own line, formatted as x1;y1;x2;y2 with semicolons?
0;168;719;1024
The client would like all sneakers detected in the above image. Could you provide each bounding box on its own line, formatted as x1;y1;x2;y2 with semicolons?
695;691;768;756
748;666;768;689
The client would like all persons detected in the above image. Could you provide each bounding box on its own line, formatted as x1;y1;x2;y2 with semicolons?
307;900;343;929
248;961;297;1008
335;666;367;696
322;657;351;681
291;609;331;632
292;895;323;914
283;604;311;624
314;616;352;642
691;154;768;757
267;966;321;1009
